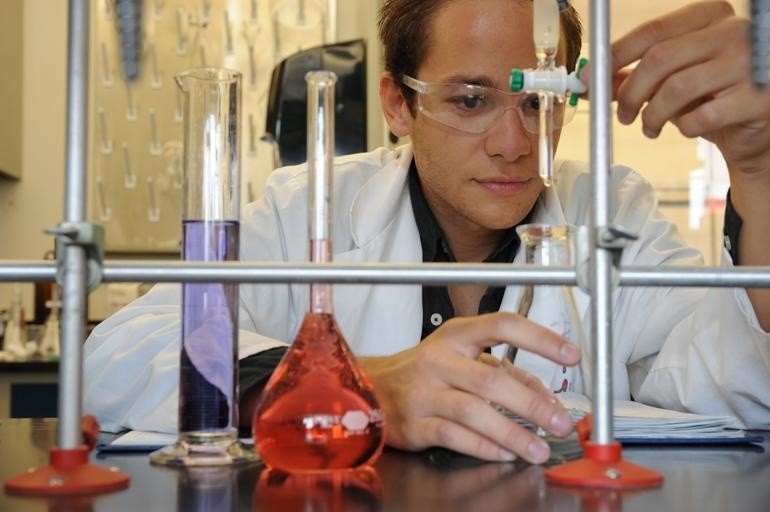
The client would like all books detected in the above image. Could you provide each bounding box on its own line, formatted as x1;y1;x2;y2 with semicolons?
100;393;763;449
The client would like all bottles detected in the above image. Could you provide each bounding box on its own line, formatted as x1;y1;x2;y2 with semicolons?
2;282;63;362
485;222;592;441
254;69;387;472
150;65;263;471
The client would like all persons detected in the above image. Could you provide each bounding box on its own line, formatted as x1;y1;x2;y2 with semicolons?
82;0;768;443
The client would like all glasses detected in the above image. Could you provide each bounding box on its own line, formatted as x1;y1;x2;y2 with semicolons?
395;71;579;135
